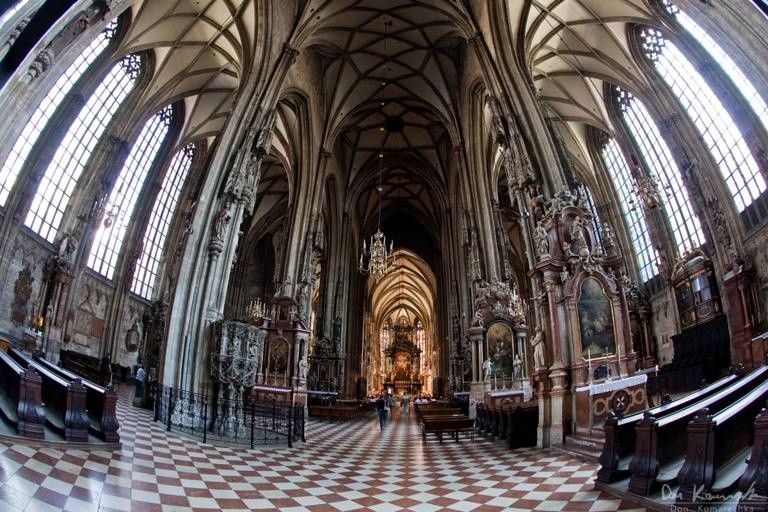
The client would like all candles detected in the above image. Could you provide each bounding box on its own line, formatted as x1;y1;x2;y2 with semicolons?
491;369;517;387
587;343;627;369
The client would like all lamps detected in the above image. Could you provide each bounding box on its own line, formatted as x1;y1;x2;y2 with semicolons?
357;19;397;283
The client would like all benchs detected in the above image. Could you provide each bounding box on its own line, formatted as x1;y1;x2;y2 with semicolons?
307;398;476;446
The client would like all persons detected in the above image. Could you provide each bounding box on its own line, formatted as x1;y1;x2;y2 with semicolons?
482;355;494;383
601;221;613;242
134;366;147;397
561;241;572;255
511;354;524;381
373;396;388;431
364;392;429;415
569;214;586;249
97;352;113;386
532;220;551;259
529;325;548;368
